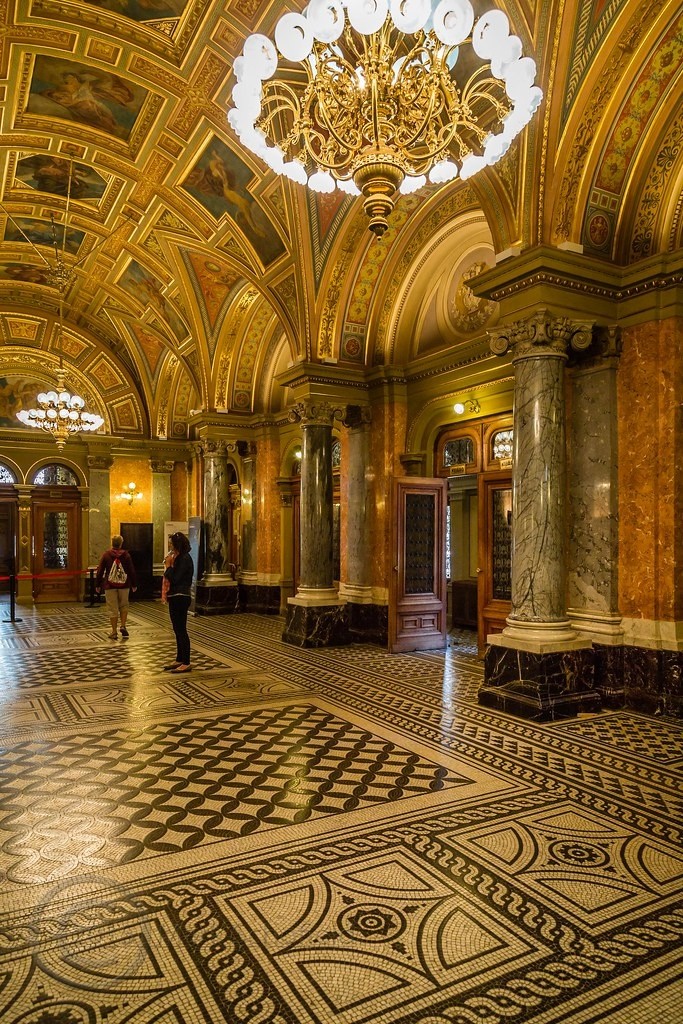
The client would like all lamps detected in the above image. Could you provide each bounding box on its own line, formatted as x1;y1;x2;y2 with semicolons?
226;0;544;241
454;399;479;414
116;482;143;504
17;212;104;452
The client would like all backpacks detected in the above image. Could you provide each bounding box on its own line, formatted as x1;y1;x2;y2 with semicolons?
107;558;127;587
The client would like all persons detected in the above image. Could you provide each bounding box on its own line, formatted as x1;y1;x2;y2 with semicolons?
95;535;137;639
160;531;194;673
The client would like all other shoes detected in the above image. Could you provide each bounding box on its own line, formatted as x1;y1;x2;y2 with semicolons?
164;664;181;670
171;665;191;673
108;633;117;639
120;625;129;636
